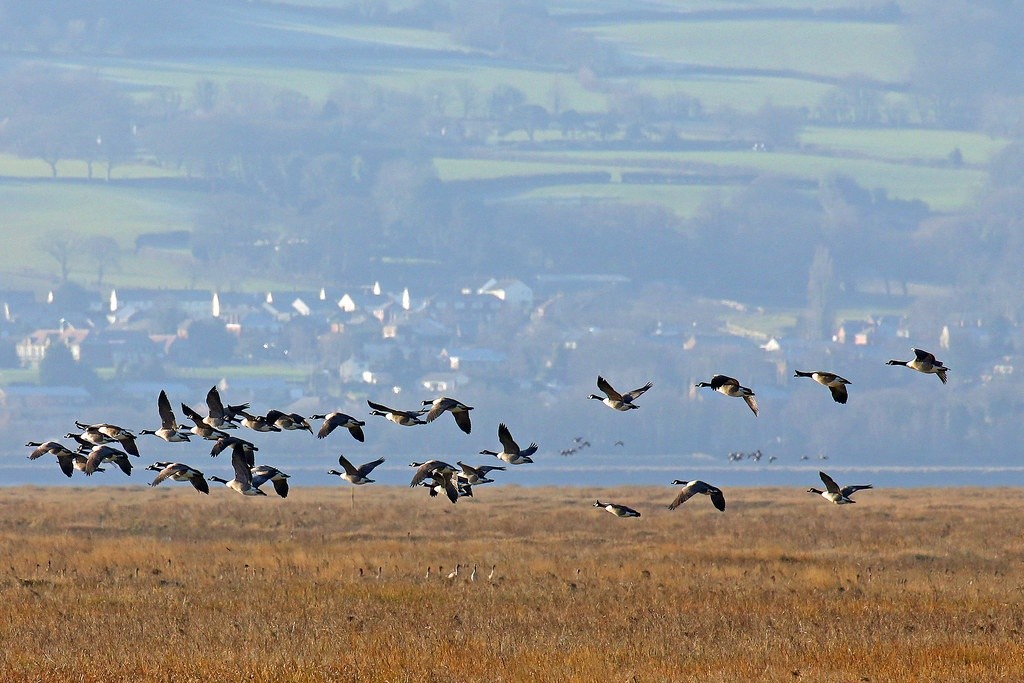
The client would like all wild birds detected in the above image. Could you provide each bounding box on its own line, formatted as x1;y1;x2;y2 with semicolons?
138;389;191;442
327;455;385;485
794;370;852;404
25;421;140;478
181;385;313;443
244;564;265;578
409;460;507;503
592;500;641;518
666;480;726;512
425;563;496;582
696;375;759;417
807;471;873;505
367;400;430;426
309;411;365;443
558;437;623;455
355;566;382;579
728;449;828;463
885;347;951;385
420;397;474;435
586;375;653;411
144;461;209;495
208;436;291;499
479;422;538;465
